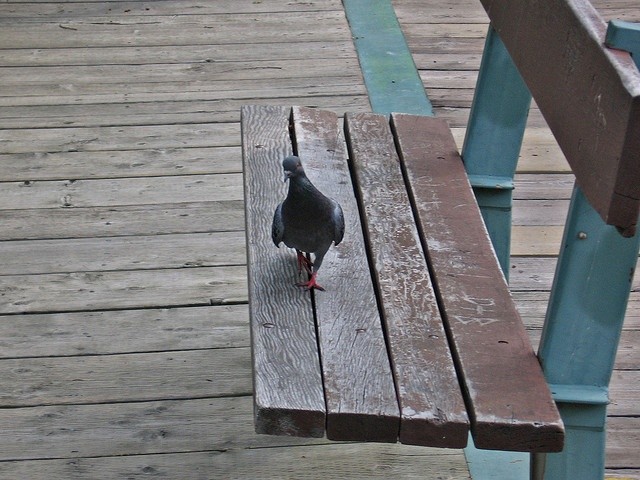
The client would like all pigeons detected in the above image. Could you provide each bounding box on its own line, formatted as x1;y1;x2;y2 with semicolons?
271;155;346;293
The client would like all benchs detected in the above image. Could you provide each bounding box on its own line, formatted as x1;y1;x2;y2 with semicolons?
241;0;640;478
